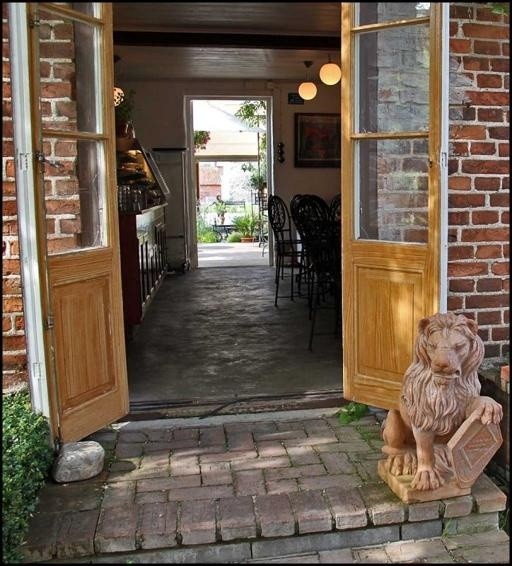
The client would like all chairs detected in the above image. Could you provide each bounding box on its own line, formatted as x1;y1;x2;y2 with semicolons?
268;191;342;352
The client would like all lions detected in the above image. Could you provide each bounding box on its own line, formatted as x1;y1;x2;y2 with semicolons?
379;310;504;492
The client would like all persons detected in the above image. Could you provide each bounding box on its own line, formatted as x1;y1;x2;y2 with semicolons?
206;195;227;224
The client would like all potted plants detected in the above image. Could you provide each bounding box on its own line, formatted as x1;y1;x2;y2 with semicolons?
230;213;260;242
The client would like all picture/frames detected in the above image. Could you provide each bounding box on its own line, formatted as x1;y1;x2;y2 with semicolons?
293;110;341;169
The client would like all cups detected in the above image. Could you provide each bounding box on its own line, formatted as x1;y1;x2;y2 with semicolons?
117;182;146;213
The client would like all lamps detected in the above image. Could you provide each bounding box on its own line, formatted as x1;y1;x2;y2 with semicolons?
319;50;342;85
114;55;126;107
298;60;318;101
